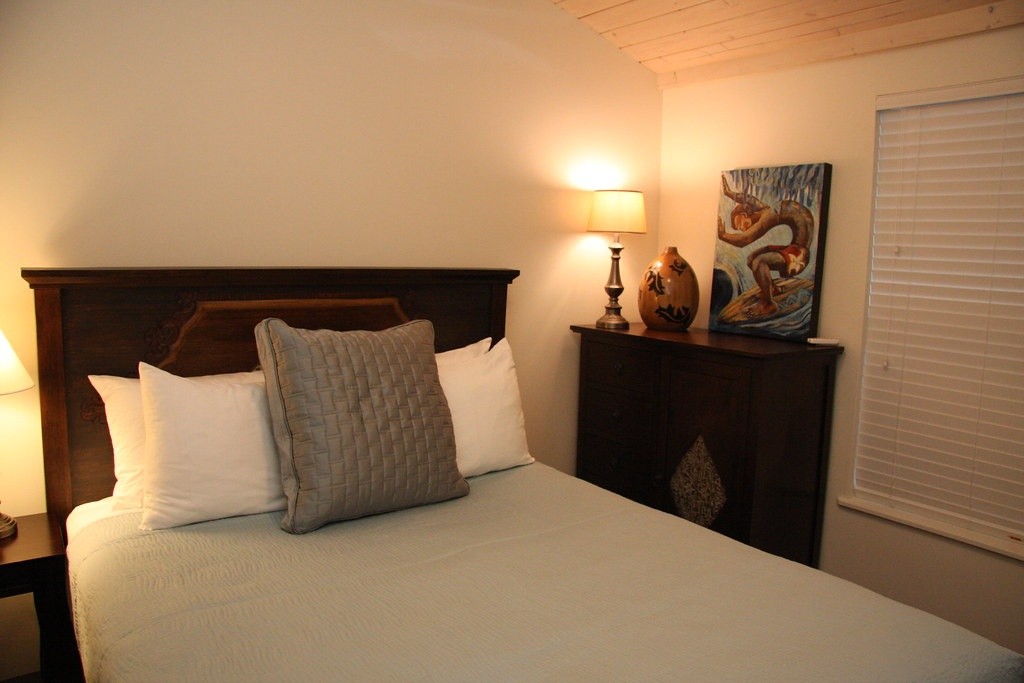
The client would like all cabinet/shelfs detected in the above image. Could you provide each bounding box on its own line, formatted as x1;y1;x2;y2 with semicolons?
570;321;845;572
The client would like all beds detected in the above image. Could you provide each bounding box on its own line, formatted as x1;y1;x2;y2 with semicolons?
18;264;1024;683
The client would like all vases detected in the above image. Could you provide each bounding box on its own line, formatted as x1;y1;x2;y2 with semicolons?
638;247;699;331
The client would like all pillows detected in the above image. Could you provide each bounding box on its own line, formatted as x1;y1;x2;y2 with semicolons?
435;338;535;477
86;360;288;532
254;316;470;536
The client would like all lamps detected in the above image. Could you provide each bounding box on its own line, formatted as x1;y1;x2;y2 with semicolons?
0;327;35;539
588;190;647;330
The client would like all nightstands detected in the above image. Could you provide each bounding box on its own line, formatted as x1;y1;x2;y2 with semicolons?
0;511;77;683
